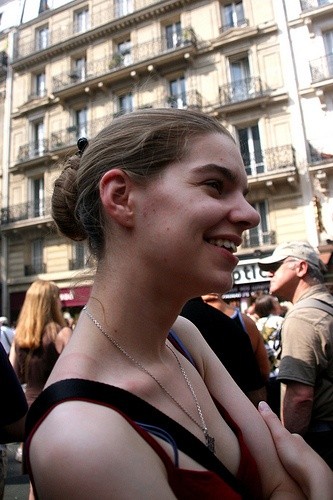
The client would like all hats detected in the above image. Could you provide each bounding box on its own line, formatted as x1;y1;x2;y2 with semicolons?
257;239;319;272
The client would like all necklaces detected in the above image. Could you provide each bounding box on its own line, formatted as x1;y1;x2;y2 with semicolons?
83;305;215;455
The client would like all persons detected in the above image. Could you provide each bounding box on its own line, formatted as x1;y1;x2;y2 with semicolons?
0;317;15;357
63;311;77;332
0;342;28;446
9;278;72;500
257;240;333;473
21;109;333;500
181;291;295;422
